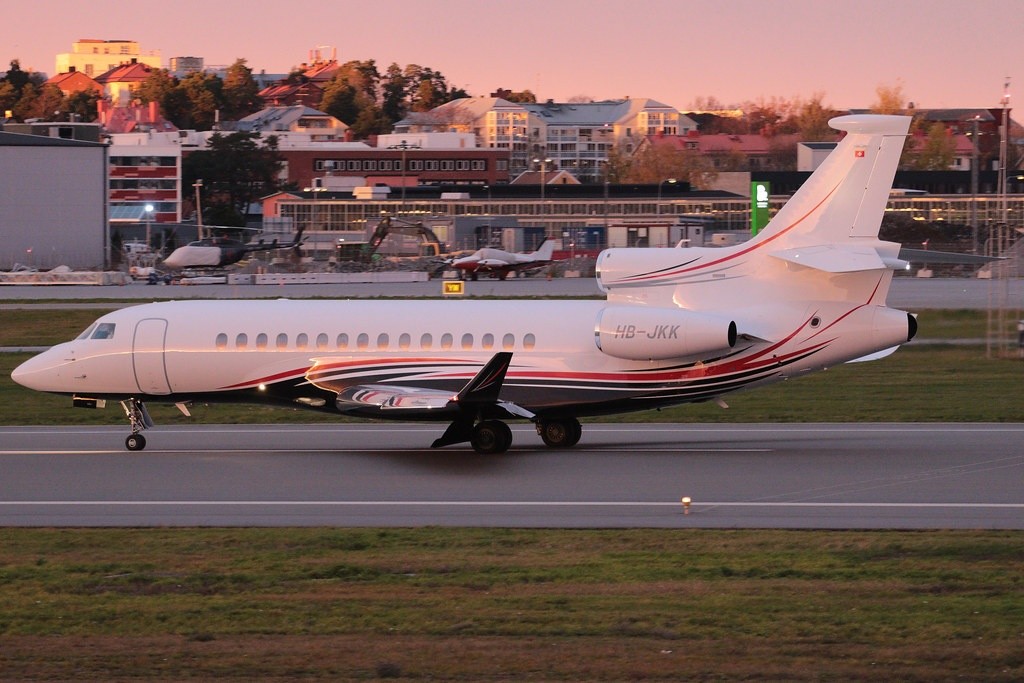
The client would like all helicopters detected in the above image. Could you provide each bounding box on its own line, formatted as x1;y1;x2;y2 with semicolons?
164;220;309;275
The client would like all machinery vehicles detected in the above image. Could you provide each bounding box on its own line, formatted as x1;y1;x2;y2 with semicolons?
362;211;450;259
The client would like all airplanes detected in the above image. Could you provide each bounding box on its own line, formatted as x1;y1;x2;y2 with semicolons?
10;110;918;455
452;236;556;279
